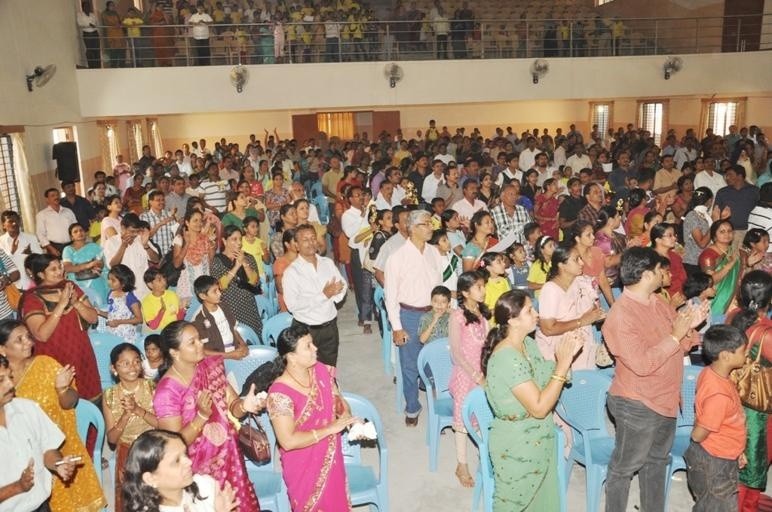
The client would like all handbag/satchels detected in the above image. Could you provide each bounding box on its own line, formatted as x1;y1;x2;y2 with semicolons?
5;284;21;311
161;233;185;286
237;265;262;294
75;262;100;280
238;422;271;466
335;396;344;420
730;356;772;413
364;251;376;272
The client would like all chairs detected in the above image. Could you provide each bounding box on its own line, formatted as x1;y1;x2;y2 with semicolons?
67;160;739;512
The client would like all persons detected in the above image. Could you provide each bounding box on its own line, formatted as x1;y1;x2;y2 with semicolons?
77;1;646;70
297;119;771;512
0;128;362;512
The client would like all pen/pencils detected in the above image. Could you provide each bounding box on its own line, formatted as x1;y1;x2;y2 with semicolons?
54;457;82;465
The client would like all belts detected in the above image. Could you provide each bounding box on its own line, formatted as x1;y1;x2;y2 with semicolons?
401;303;433;311
293;318;337;328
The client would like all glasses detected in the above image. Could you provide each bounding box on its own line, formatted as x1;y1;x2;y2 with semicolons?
116;359;140;367
417;222;434;227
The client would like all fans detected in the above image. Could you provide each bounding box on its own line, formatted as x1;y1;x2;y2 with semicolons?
230;63;249;92
384;62;403;87
664;56;684;79
529;59;549;84
26;62;56;91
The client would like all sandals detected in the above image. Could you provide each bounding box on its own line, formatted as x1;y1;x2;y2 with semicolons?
456;470;475;487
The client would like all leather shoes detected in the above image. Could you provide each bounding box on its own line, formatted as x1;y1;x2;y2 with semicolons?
406;417;418;426
358;316;379;334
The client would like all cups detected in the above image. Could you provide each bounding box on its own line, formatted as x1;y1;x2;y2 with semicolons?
463;256;474;272
